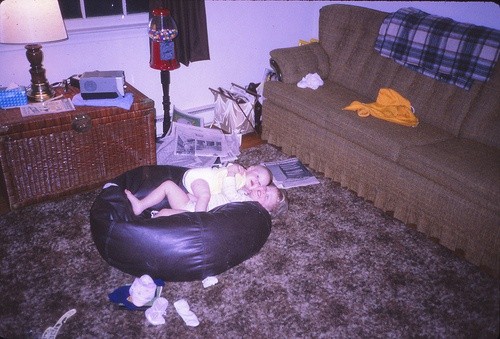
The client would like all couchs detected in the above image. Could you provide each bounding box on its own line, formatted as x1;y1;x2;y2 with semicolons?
260;4;500;279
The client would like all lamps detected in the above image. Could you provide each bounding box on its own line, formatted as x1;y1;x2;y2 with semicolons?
0;0;69;103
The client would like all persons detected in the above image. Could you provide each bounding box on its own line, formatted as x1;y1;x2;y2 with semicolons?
125;180;289;218
182;163;272;212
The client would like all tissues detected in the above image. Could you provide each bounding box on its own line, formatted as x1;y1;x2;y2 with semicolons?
0;82;29;109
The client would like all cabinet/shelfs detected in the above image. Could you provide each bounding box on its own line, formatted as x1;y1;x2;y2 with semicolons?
0;82;157;213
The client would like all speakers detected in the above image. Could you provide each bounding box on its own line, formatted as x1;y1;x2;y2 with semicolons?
79;70;125;101
